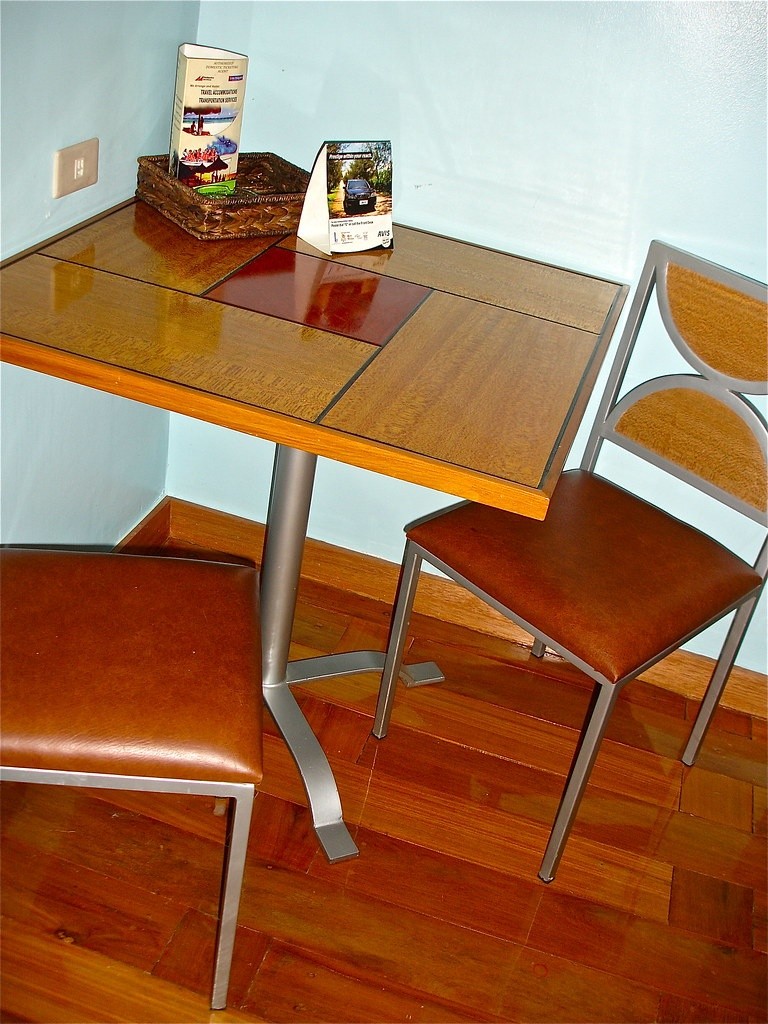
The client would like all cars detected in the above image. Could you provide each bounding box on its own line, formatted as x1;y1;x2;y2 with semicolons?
342;178;377;213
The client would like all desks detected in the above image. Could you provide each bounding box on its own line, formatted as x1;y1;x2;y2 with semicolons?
0;199;632;866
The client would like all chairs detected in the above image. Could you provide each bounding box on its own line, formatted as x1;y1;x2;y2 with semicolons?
374;237;767;881
0;547;262;1012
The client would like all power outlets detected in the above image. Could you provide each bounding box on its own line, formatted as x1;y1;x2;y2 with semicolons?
54;137;99;200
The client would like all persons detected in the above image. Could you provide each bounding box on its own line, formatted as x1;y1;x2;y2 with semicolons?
181;146;217;163
210;172;226;184
191;121;196;132
200;117;204;128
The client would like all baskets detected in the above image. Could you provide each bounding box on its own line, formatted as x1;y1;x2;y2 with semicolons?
136;153;313;240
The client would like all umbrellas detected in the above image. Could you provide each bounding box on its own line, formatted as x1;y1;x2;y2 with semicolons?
192;163;210;181
206;154;228;176
184;106;221;135
